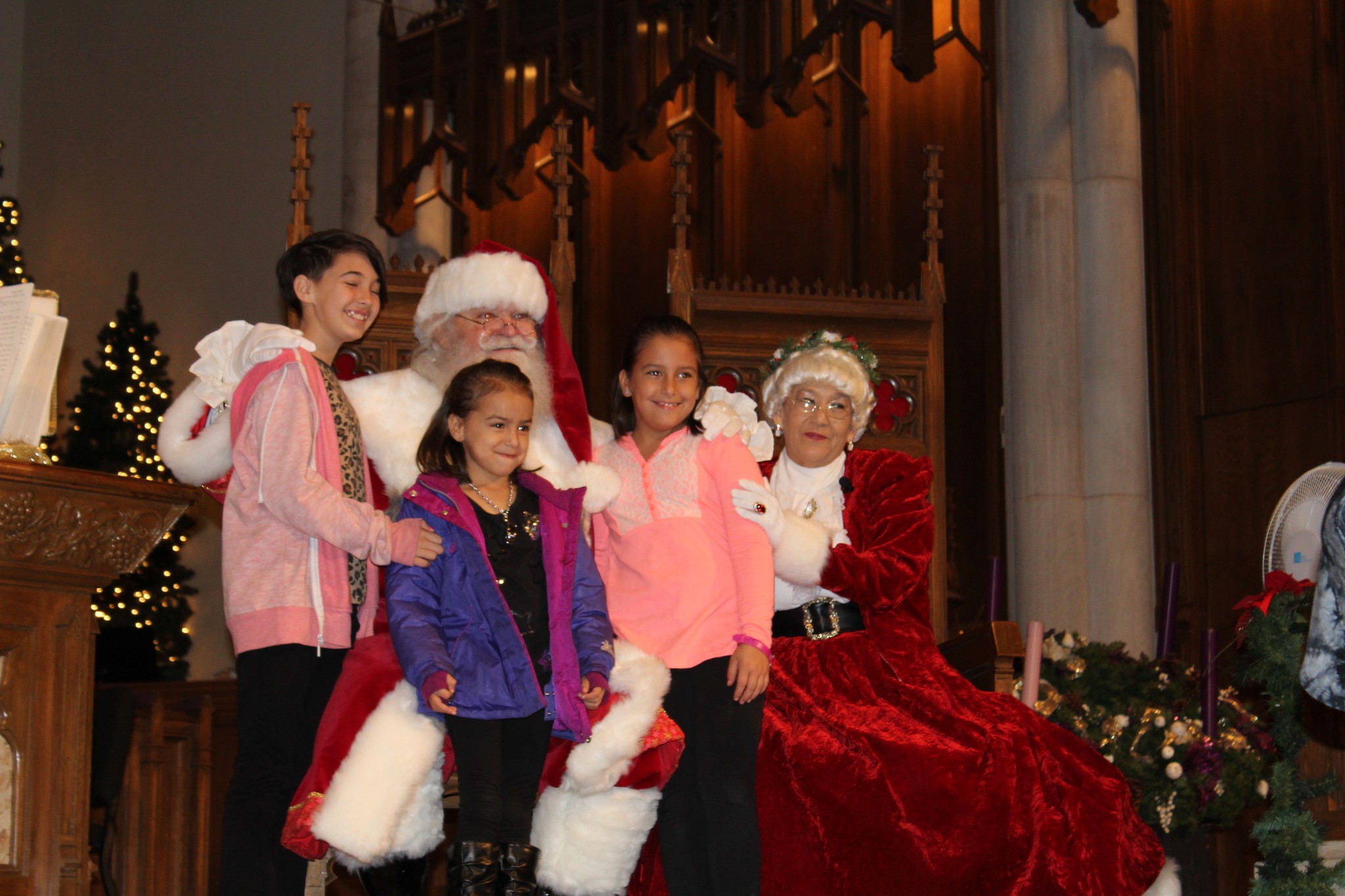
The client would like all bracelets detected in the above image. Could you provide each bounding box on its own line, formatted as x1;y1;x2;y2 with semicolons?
732;636;773;668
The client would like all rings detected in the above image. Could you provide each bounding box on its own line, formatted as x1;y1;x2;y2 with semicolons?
753;502;766;514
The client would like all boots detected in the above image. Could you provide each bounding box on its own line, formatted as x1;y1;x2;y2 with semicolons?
459;841;501;896
502;842;541;896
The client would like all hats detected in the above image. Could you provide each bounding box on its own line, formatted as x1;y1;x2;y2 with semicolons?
416;240;623;513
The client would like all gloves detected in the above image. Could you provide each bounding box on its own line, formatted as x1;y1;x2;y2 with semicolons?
223;322;317;383
694;401;751;446
731;476;785;549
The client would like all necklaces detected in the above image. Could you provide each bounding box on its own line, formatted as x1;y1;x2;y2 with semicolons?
466;477;515;546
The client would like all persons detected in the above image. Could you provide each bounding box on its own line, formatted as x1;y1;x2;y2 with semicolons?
338;239;1182;896
217;229;444;896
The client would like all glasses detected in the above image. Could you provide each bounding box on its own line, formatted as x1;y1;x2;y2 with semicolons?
786;396;854;420
455;314;536;336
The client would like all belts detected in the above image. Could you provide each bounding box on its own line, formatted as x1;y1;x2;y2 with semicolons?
771;597;866;640
